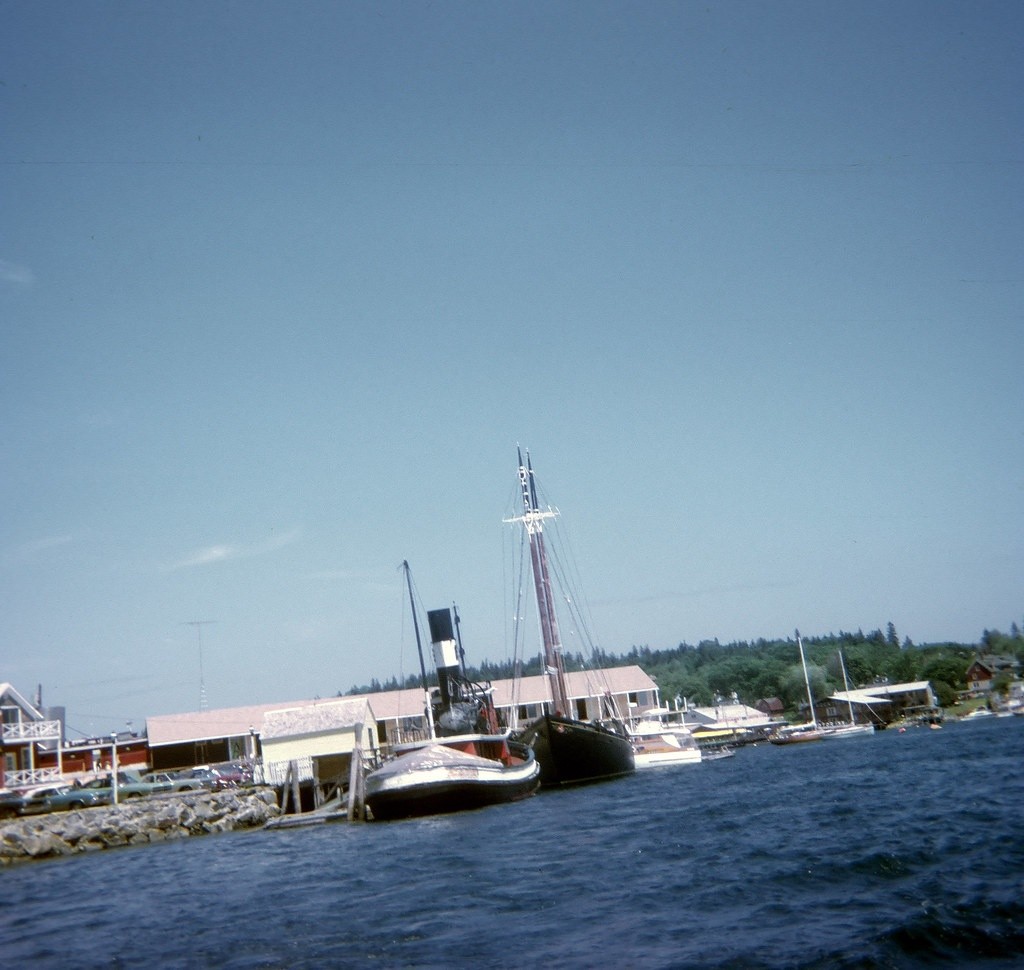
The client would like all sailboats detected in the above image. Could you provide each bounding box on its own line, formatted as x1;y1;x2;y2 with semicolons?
819;651;874;740
365;560;540;819
769;637;825;745
515;445;636;790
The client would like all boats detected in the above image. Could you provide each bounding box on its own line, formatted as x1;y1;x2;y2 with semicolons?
960;710;992;722
626;729;701;770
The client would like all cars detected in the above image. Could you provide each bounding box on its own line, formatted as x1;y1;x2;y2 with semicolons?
141;772;213;791
0;793;52;817
80;776;172;802
174;764;252;792
32;785;114;811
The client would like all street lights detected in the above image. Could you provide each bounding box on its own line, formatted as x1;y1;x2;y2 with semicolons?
248;725;258;757
111;732;118;804
